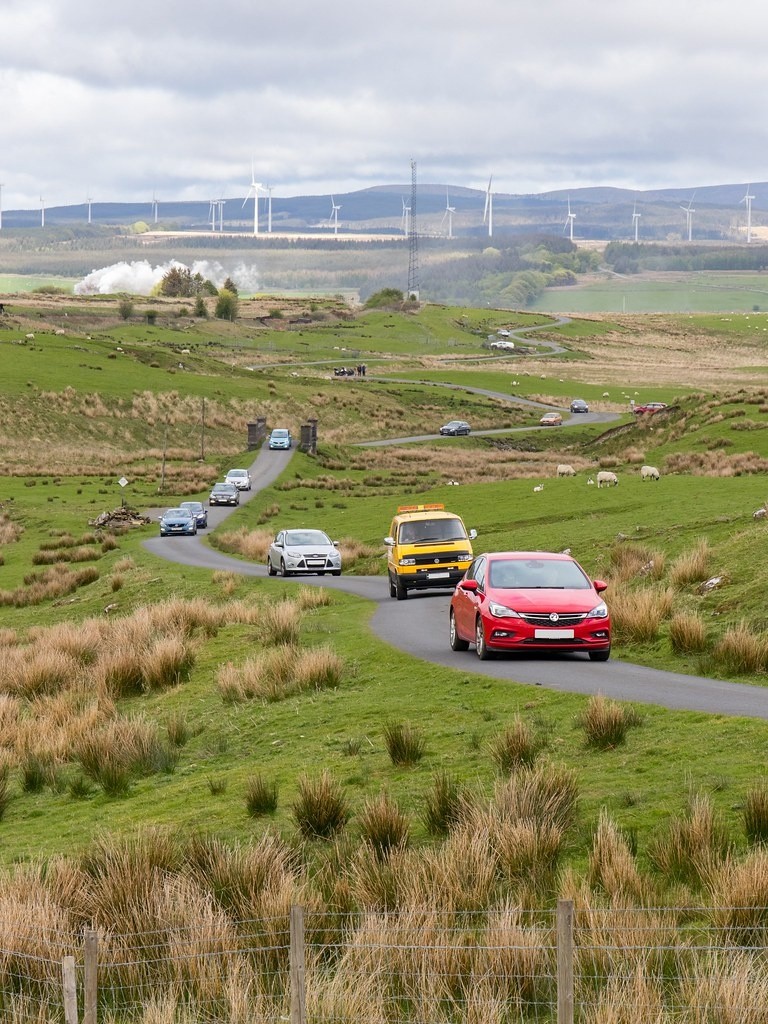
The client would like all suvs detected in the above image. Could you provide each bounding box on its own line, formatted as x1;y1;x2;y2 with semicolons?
268;428;292;450
633;403;668;417
490;341;514;351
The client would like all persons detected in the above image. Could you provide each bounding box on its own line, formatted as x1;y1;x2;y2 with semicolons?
347;364;366;376
402;525;421;541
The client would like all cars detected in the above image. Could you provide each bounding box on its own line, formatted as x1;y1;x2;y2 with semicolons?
539;413;563;427
158;508;198;537
570;400;589;413
179;502;208;529
266;529;342;577
449;551;613;662
439;420;472;436
208;482;240;507
496;330;510;337
224;469;253;491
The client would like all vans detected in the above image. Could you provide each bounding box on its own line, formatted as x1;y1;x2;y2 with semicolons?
383;503;478;600
